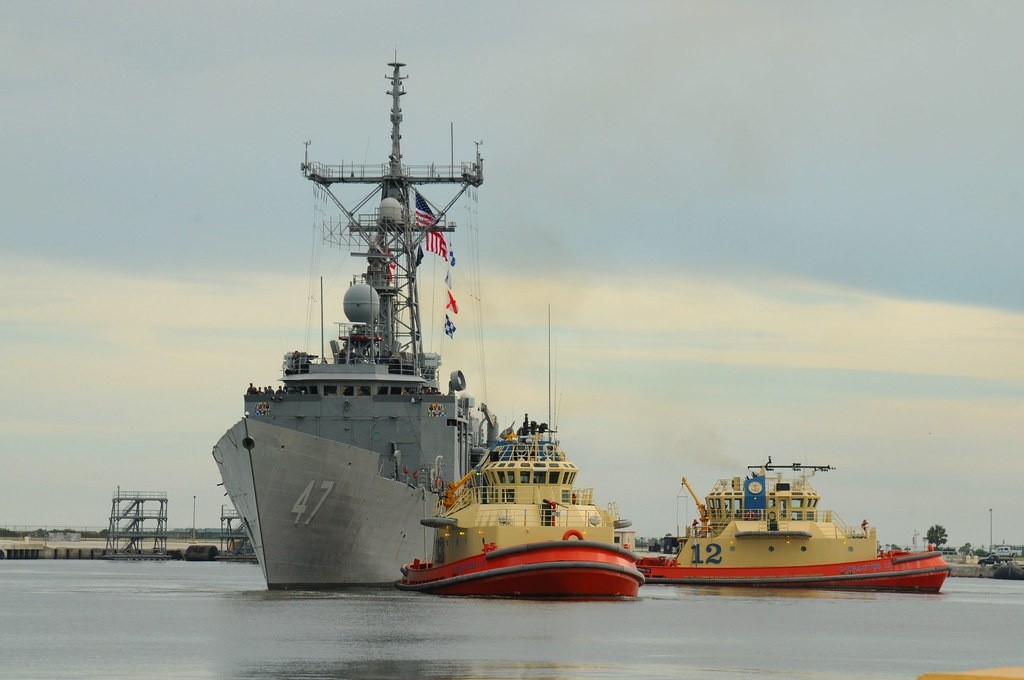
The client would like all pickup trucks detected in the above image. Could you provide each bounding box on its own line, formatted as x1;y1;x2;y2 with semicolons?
978;554;1012;566
996;547;1022;557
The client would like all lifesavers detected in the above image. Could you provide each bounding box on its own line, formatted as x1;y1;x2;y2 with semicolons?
563;529;584;540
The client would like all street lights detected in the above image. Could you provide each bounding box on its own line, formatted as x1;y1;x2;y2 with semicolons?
989;508;992;554
192;496;197;540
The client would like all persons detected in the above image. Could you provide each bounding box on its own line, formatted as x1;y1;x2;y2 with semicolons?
379;351;392;363
692;519;699;537
246;383;299;395
402;387;432;395
338;350;346;361
292;351;310;363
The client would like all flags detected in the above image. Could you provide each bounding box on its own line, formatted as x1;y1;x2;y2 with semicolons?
415;192;447;260
446;291;458;314
387;247;396;280
444;315;456;338
449;244;455;266
445;271;451;288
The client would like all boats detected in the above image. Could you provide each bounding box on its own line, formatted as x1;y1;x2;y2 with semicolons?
394;413;645;598
634;455;952;594
212;51;516;592
